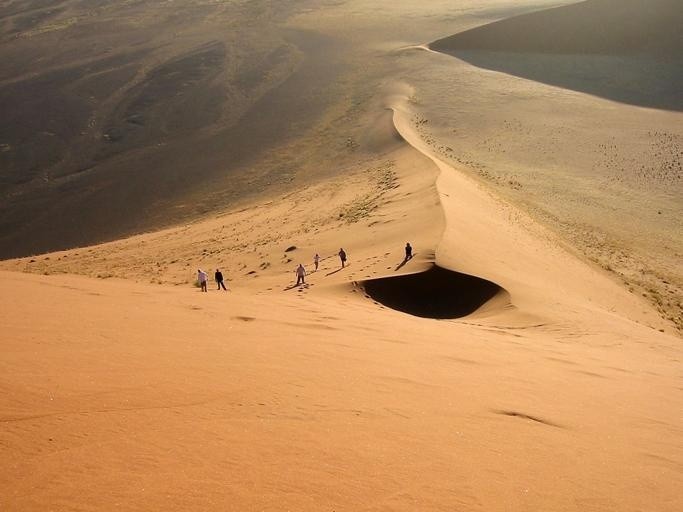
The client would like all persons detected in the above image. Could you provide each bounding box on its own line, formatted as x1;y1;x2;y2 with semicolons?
405;242;411;261
214;269;226;291
312;253;320;272
196;268;208;292
294;263;305;286
338;248;346;268
